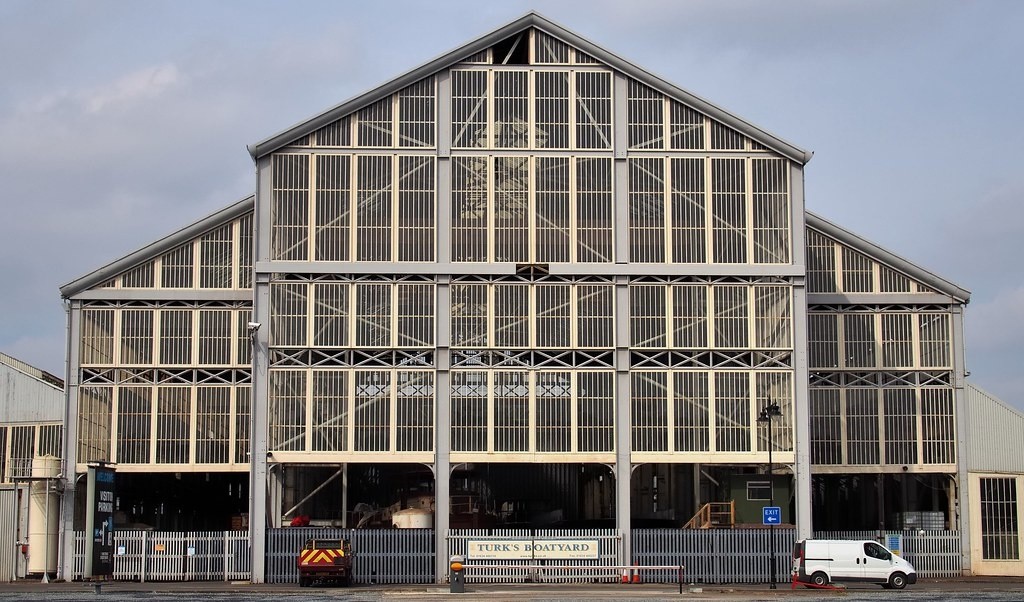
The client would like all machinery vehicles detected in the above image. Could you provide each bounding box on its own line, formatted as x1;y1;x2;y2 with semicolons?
298;539;356;587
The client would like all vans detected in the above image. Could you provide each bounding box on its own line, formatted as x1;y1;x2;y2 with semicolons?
791;540;917;589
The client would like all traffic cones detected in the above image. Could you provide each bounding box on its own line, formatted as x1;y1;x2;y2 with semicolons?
630;561;643;584
622;568;630;584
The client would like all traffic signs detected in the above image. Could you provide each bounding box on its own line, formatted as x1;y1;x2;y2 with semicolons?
763;507;782;525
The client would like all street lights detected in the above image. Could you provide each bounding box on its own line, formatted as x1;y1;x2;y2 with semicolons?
756;394;784;590
